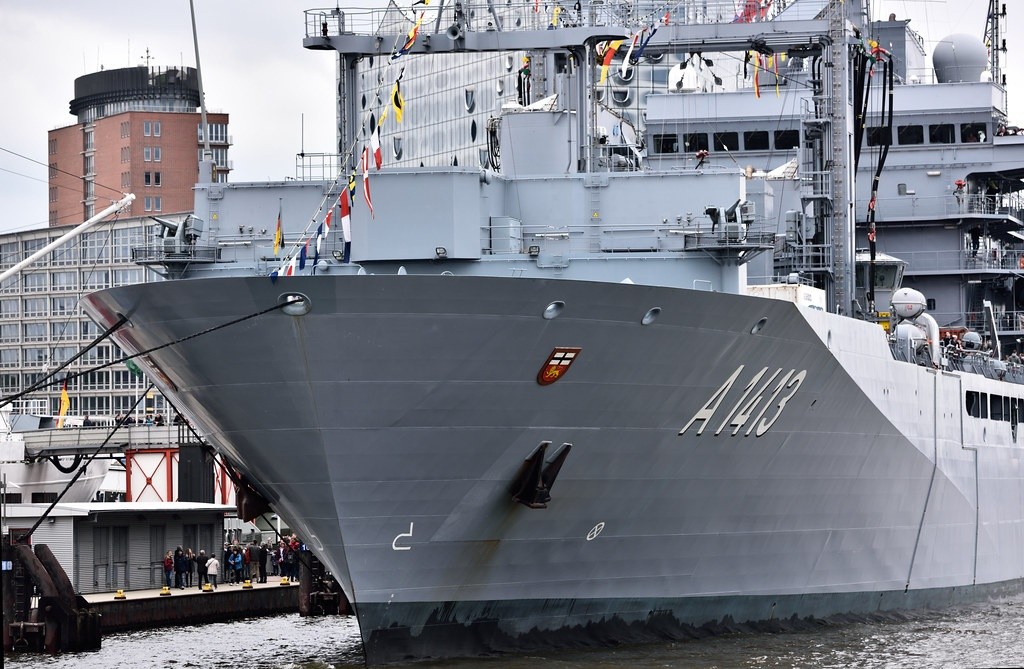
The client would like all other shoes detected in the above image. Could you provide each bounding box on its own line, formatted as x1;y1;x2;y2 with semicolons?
295;580;300;582
190;585;192;587
199;588;202;590
287;577;289;582
235;582;239;586
258;580;267;583
180;586;184;589
229;583;234;586
291;580;294;582
215;588;218;590
250;579;253;583
185;585;189;588
257;580;260;583
280;574;285;577
224;580;231;583
168;586;173;588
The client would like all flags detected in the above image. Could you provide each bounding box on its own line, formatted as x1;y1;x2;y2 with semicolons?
274;210;285;256
56;370;70;428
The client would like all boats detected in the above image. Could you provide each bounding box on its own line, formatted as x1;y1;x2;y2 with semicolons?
77;2;1024;669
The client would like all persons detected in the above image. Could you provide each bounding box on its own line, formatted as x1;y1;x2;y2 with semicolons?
995;123;1024;137
985;184;1001;214
963;130;987;143
953;187;965;214
224;539;267;586
143;414;164;426
968;224;984;261
941;332;963;363
83;416;91;430
115;412;143;428
173;413;189;426
174;545;197;590
270;533;304;582
197;549;219;590
1002;350;1024;365
163;550;174;590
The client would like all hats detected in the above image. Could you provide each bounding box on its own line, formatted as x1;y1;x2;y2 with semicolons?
176;546;182;551
210;553;216;558
233;546;238;551
